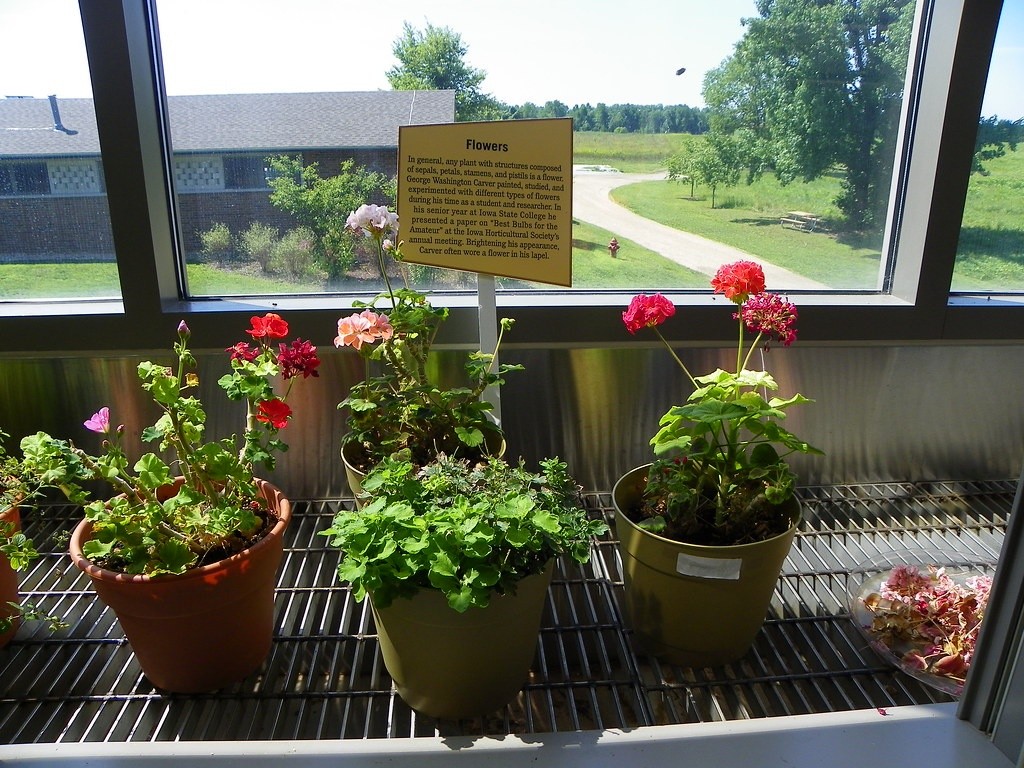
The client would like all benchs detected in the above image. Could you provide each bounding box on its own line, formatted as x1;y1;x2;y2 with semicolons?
780;217;807;231
801;217;828;231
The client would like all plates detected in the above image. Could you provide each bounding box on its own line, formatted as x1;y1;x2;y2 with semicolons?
845;547;1001;697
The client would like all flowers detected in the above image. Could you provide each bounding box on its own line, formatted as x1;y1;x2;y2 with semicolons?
20;312;321;581
622;260;828;543
856;560;992;695
333;204;525;468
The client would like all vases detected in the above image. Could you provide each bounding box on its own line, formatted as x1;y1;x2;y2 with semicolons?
69;475;292;694
612;463;802;670
340;430;506;511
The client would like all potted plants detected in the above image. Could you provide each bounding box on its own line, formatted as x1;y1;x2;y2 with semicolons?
317;438;610;721
0;426;77;649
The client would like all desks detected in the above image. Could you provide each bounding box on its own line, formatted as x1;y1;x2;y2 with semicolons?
787;210;822;232
